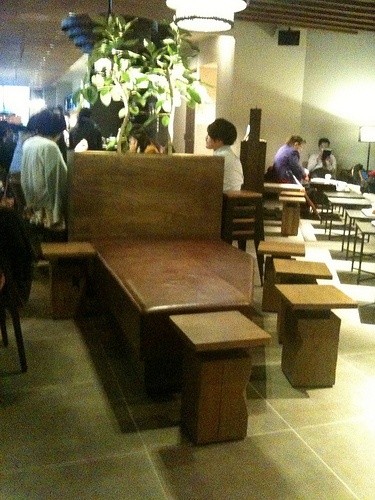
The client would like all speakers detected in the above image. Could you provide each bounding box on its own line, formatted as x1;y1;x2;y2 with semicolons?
278;30;300;45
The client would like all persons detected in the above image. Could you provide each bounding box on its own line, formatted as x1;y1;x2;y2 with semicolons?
206;118;245;192
272;135;323;215
0;107;103;311
127;126;162;155
307;138;338;205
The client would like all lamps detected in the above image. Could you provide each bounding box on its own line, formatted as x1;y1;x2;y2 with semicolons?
166;0;251;33
60;0;160;58
358;125;375;174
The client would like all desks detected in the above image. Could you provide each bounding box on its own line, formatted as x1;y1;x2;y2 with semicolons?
311;176;375;285
84;235;254;400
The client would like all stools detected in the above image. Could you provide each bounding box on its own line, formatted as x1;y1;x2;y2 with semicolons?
167;310;272;447
0;302;28;374
40;241;97;320
272;257;333;342
220;181;307;285
257;240;307;312
274;283;359;389
